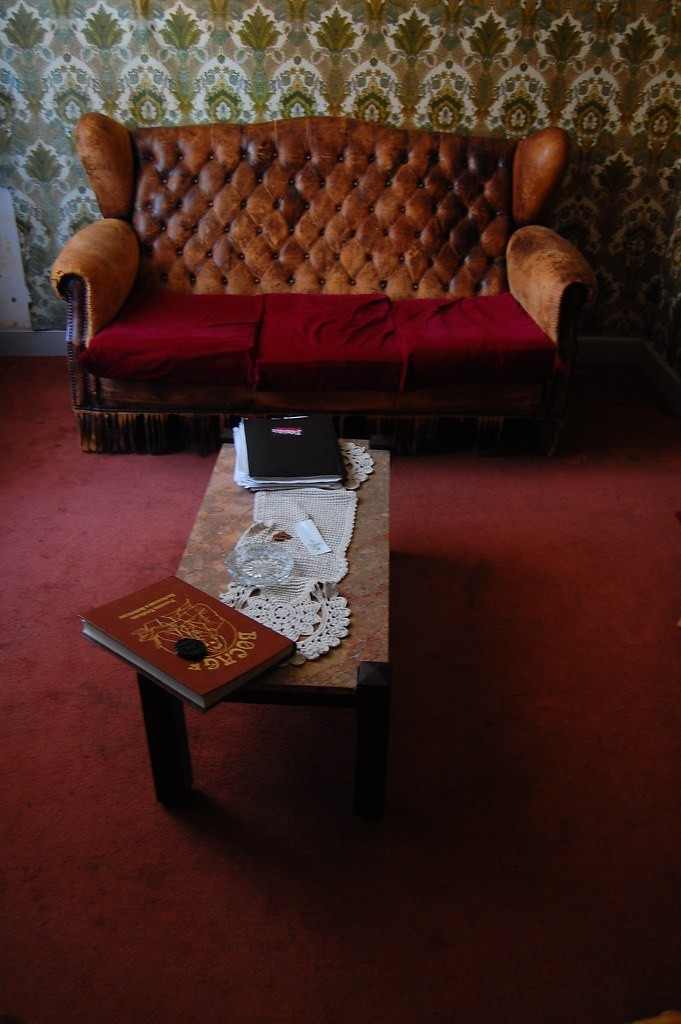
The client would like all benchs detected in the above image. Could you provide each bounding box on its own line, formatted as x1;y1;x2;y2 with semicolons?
49;112;598;456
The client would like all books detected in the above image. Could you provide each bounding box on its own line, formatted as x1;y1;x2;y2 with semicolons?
77;576;298;716
233;415;348;492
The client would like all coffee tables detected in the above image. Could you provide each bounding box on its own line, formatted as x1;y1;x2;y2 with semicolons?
137;443;395;820
